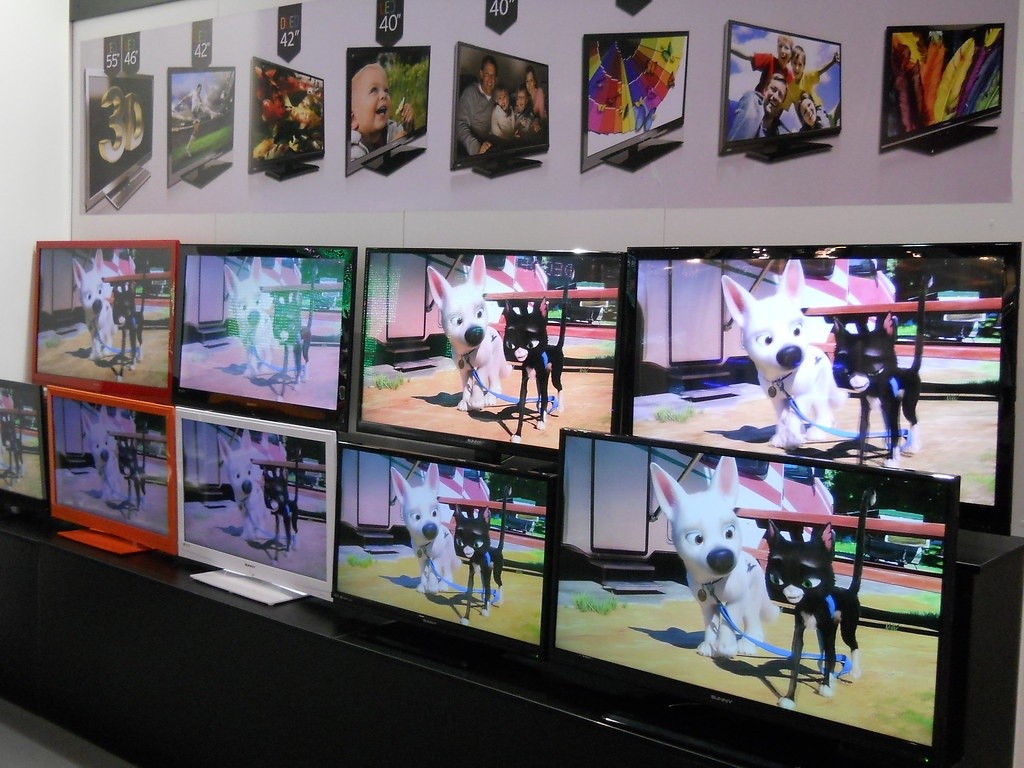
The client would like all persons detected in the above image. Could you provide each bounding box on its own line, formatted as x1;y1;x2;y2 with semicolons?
176;83;220;160
510;87;540;142
452;54;499;155
349;63;415;162
730;33;796;93
524;65;549;128
727;73;788;143
490;83;515;141
780;44;840;124
797;92;841;132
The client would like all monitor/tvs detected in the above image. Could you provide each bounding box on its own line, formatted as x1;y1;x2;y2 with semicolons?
580;30;690;173
83;68;154;212
247;56;326;180
0;239;1020;768
718;19;842;164
167;66;236;191
879;23;1005;157
345;46;431;177
450;41;549;178
